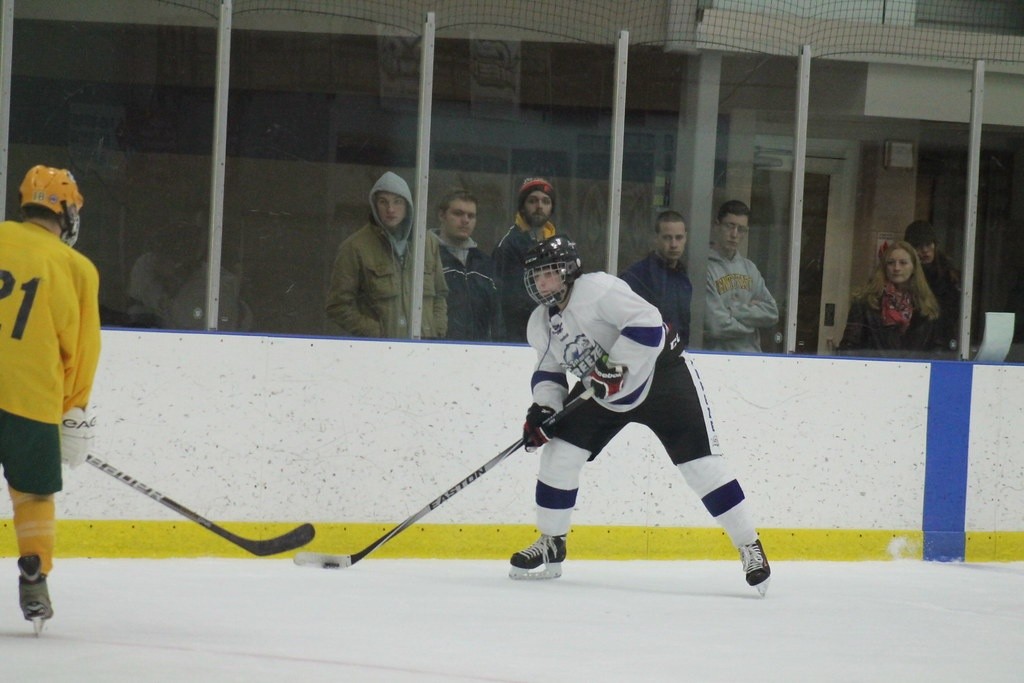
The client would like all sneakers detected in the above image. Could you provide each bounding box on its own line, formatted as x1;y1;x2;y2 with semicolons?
18;553;55;634
739;539;770;595
510;533;568;579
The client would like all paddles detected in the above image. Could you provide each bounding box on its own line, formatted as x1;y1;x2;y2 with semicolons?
86;454;316;557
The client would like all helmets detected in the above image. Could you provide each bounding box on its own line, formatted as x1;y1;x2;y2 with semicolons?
522;232;581;307
20;165;83;248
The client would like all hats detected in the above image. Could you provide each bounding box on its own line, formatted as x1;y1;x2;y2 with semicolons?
904;219;941;249
518;178;556;211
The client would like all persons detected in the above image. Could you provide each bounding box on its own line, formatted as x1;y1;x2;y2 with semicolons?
705;200;778;352
618;210;693;350
326;170;446;340
428;190;506;346
0;165;102;636
903;222;958;350
491;176;564;343
510;232;768;601
838;240;939;359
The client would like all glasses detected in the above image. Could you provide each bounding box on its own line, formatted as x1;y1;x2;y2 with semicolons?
723;222;749;234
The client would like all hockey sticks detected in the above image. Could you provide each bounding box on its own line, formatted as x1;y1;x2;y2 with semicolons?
293;386;595;569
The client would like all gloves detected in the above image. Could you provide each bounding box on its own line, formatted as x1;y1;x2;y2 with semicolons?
62;408;97;472
590;364;622;399
522;405;554;445
